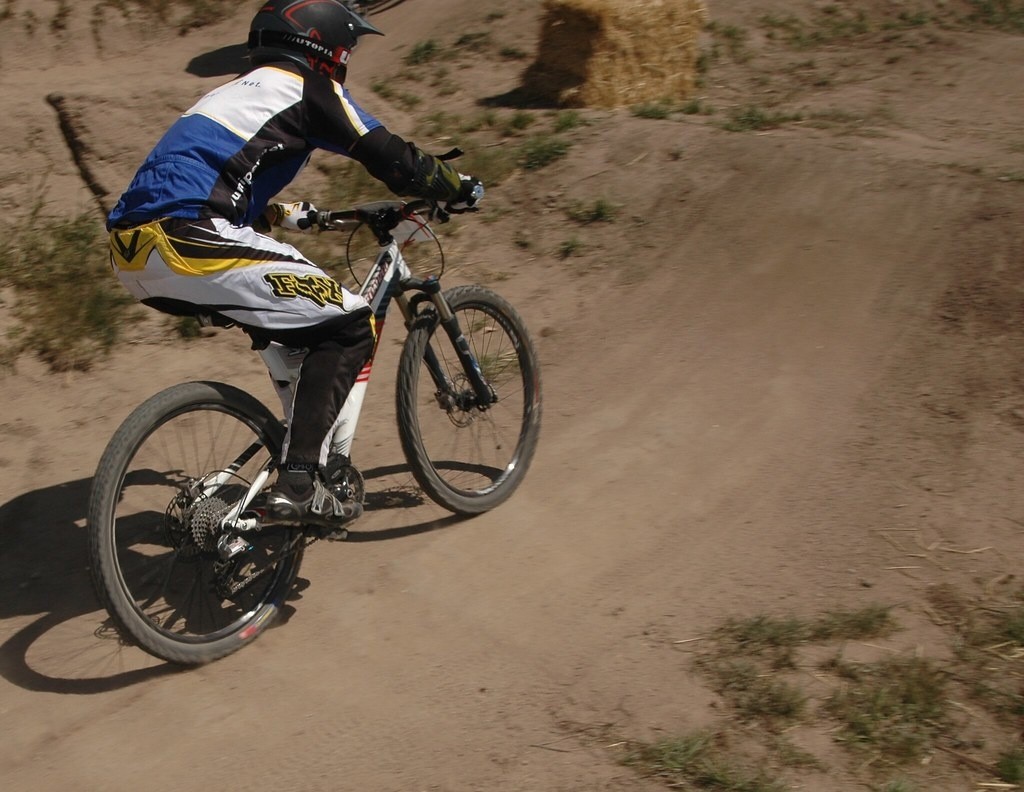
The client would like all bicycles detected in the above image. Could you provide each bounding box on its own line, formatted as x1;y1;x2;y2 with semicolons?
82;146;542;666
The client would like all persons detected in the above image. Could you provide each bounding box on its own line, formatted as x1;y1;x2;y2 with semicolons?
106;0;483;527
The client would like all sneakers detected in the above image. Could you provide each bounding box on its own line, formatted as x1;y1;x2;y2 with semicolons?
266;473;363;526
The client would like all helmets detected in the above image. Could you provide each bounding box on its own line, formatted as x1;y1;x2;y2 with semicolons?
246;0;383;86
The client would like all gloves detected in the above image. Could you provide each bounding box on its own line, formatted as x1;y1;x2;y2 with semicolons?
274;201;319;234
434;173;483;215
